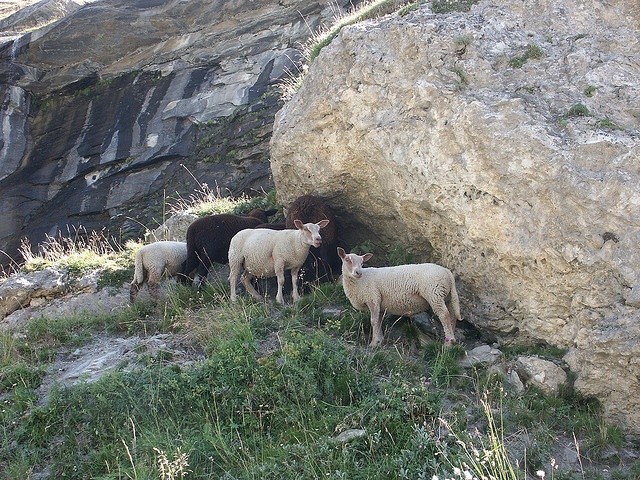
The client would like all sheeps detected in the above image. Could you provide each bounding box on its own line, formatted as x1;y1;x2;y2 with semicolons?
251;223;286;299
130;241;189;307
287;195;335;295
228;220;330;304
182;209;279;290
336;247;463;349
